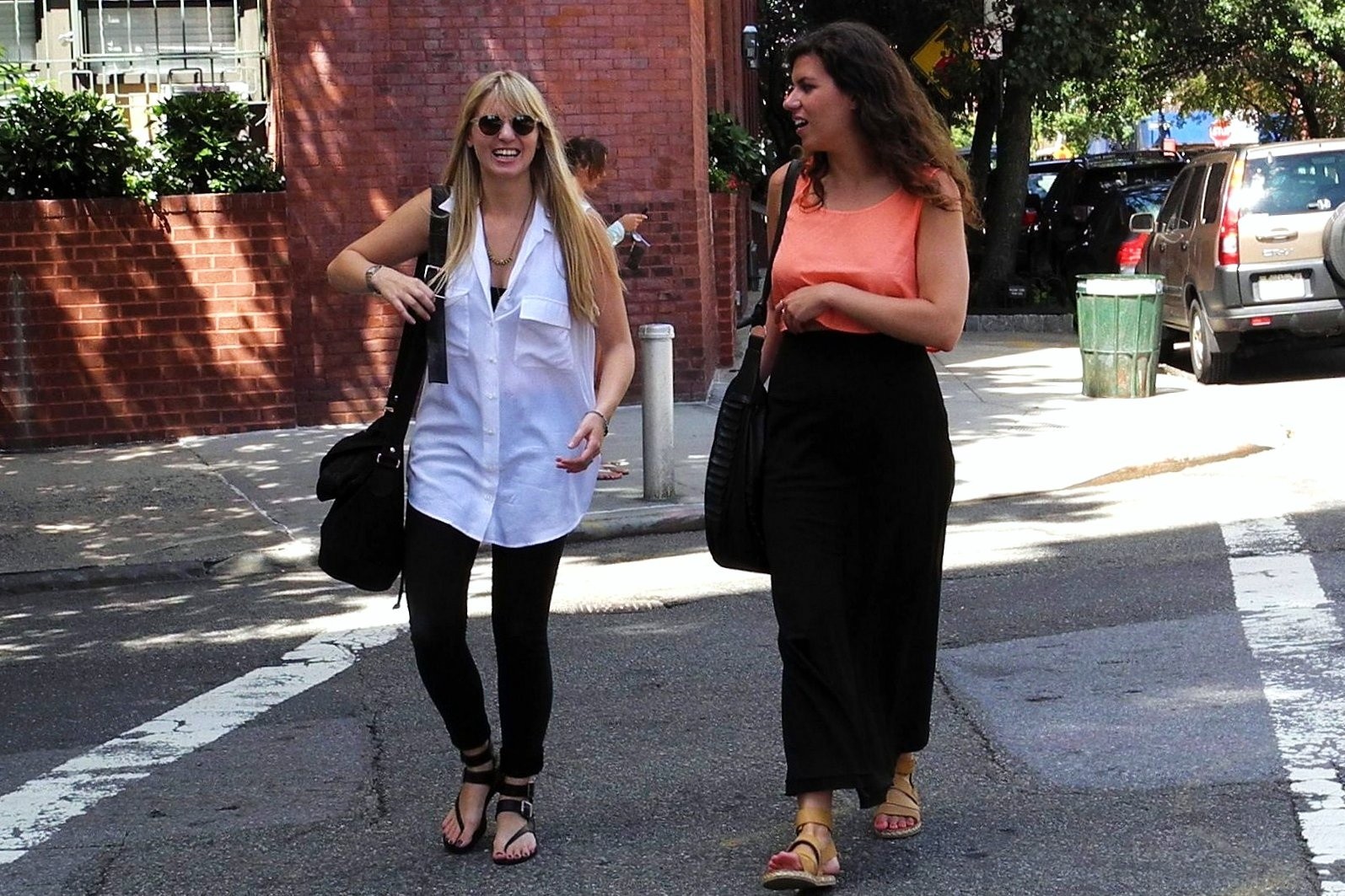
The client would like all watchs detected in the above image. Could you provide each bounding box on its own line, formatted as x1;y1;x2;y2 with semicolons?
365;264;385;295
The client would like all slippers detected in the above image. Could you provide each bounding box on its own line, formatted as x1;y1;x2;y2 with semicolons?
599;463;630;479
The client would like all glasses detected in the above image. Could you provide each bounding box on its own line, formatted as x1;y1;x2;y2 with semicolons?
470;111;537;137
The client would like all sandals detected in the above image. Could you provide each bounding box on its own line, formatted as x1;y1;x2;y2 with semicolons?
871;757;921;837
763;806;840;887
489;783;539;866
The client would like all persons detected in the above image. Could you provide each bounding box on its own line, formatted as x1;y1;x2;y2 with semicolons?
754;22;985;891
563;135;649;482
328;69;640;864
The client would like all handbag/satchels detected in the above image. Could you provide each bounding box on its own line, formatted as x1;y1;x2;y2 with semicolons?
702;332;769;574
311;420;416;591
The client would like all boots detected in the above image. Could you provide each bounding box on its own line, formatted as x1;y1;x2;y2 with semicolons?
442;747;495;851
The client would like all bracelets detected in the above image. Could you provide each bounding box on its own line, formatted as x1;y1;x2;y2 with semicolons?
583;410;609;437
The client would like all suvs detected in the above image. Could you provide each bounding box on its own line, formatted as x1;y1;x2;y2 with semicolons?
1028;149;1187;319
1129;135;1345;384
986;158;1073;280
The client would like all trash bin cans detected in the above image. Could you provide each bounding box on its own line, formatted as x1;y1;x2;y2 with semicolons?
1074;270;1169;396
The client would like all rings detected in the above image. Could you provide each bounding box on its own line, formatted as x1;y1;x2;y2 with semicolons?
780;299;783;307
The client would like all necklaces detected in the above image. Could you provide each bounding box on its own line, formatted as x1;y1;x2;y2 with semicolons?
476;186;536;267
